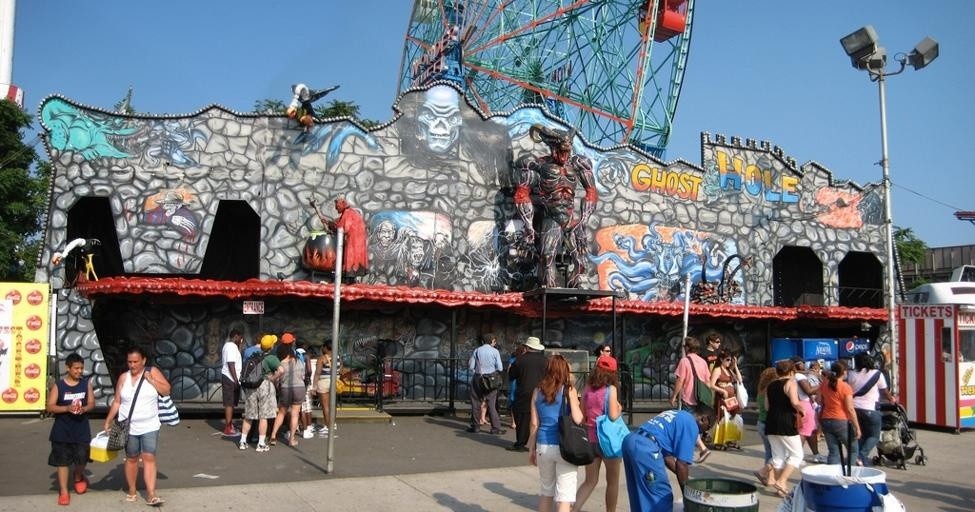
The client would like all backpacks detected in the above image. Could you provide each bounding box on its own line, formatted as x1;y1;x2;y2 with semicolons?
239;351;272;389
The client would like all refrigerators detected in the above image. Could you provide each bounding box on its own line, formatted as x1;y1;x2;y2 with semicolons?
770;337;839;370
838;336;871;368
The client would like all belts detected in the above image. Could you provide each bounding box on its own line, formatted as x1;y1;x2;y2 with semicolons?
634;428;656;441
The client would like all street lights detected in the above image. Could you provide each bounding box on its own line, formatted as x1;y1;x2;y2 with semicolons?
831;21;939;416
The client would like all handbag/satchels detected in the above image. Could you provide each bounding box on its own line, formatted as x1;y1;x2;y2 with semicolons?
478;371;503;394
694;377;712;410
158;394;180;427
106;418;130;451
724;396;738;411
596;414;631;460
336;378;345;395
559;415;594;465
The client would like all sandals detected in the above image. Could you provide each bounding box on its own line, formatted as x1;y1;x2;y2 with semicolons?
58;493;70;506
73;470;88;494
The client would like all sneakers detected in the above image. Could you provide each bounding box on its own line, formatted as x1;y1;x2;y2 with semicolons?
466;427;479;433
505;443;524;451
812;454;827;463
695;447;711;463
222;422;337;453
491;430;505;435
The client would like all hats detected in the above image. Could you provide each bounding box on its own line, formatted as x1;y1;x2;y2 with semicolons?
522;336;545;351
281;334;295;344
261;335;277;351
597;356;618;372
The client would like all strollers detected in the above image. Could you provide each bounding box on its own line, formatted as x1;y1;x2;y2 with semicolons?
868;399;928;474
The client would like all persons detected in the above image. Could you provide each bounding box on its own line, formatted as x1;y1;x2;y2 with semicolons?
571;356;623;512
595;344;611;360
755;353;900;497
467;334;504;433
47;354;95;506
55;238;101;288
507;348;526;428
103;349;171;506
478;335;496;426
528;355;584;512
509;337;549;447
322;198;369;285
622;404;716;512
670;333;743;450
220;330;340;453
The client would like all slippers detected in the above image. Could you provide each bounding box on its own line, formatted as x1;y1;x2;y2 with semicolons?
145;497;165;505
126;493;137;502
752;471;790;494
